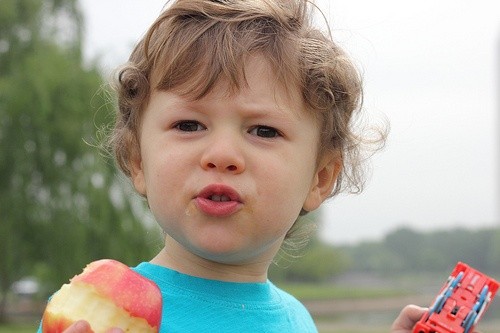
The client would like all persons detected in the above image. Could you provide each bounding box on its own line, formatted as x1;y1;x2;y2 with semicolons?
36;0;479;333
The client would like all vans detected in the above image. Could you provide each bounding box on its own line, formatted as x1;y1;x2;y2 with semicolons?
410;258;500;333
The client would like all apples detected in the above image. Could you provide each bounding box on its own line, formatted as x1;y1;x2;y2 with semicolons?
41;259;163;333
